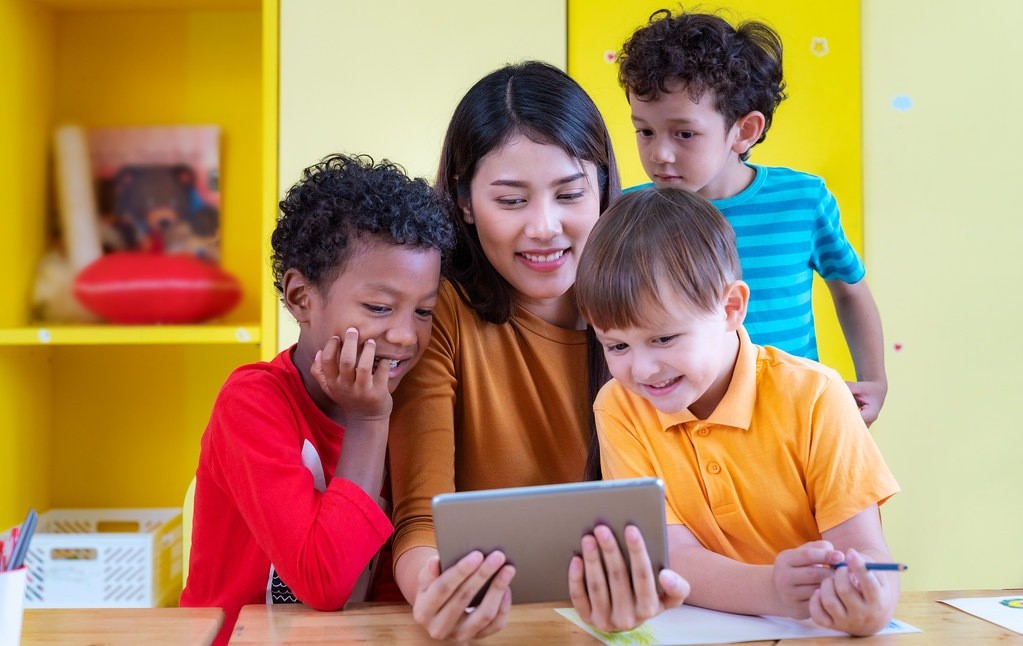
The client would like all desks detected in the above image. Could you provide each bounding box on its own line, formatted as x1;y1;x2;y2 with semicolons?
19;589;1023;646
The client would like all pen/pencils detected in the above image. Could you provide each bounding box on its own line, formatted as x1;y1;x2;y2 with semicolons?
829;562;908;571
7;510;38;569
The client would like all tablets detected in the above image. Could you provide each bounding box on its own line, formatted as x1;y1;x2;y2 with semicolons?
431;479;670;609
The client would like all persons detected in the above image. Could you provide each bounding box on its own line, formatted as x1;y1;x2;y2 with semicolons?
368;60;691;645
576;187;901;636
180;153;456;646
618;10;889;428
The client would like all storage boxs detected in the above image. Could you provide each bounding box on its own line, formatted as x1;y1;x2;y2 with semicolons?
0;509;182;609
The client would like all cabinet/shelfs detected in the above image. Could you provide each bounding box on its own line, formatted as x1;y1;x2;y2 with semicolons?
0;0;277;609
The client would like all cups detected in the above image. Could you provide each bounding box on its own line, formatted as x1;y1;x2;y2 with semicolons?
0;563;26;646
